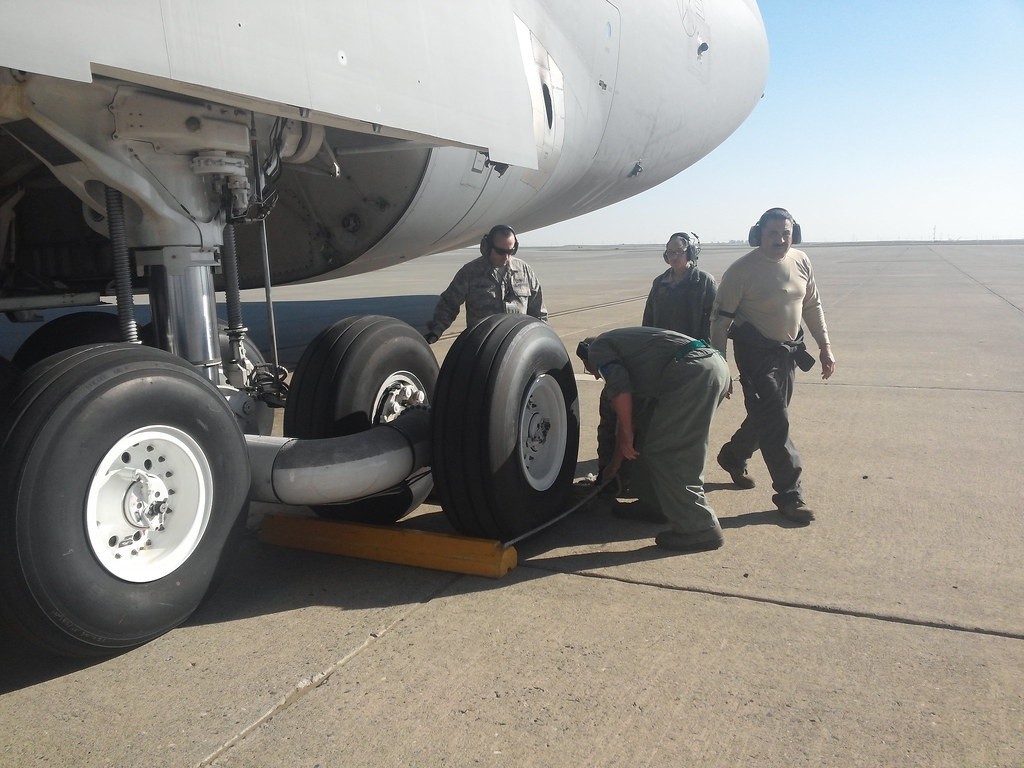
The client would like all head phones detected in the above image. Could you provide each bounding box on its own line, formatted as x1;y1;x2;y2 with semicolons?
663;232;699;262
480;224;519;256
576;342;590;360
749;207;801;246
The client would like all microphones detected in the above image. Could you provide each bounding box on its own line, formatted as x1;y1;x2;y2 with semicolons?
691;232;701;255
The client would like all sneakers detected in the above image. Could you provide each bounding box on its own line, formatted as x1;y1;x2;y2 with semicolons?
613;499;668;523
778;494;816;521
655;523;724;550
595;470;621;496
717;440;756;489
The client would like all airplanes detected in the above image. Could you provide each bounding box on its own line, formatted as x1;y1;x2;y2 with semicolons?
0;0;770;662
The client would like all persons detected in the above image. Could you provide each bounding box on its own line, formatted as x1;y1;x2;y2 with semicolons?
576;326;732;552
423;224;549;347
640;233;719;353
710;207;836;521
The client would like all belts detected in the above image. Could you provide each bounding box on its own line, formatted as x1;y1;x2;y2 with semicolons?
657;339;711;386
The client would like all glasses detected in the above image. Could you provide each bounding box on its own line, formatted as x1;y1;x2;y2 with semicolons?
489;240;515;255
667;248;687;257
